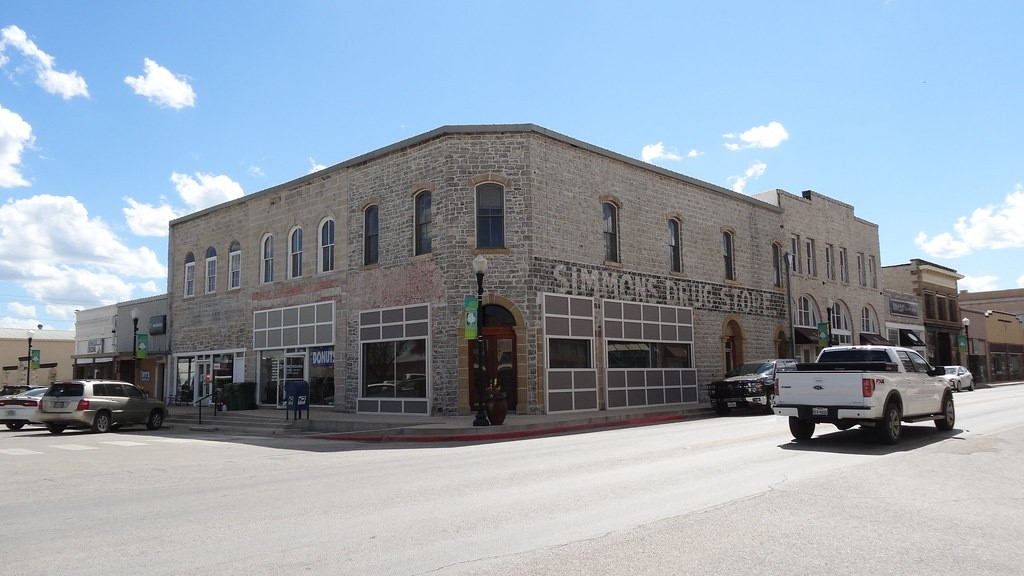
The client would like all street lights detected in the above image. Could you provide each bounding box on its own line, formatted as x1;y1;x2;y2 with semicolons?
26;329;36;386
471;252;491;427
961;316;971;374
130;308;141;384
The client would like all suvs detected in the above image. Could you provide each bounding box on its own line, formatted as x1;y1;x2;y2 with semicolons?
38;378;169;434
0;385;45;397
708;358;796;418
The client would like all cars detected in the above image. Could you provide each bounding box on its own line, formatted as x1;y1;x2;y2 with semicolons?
935;366;974;393
0;388;49;430
365;373;426;397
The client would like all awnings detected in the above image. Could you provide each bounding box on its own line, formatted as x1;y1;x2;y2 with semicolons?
860;333;894;346
900;329;926;346
794;327;838;345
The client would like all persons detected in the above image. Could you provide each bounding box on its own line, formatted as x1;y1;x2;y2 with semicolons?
182;381;189;406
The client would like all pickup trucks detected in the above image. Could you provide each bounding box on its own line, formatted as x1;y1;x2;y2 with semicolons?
770;344;956;444
